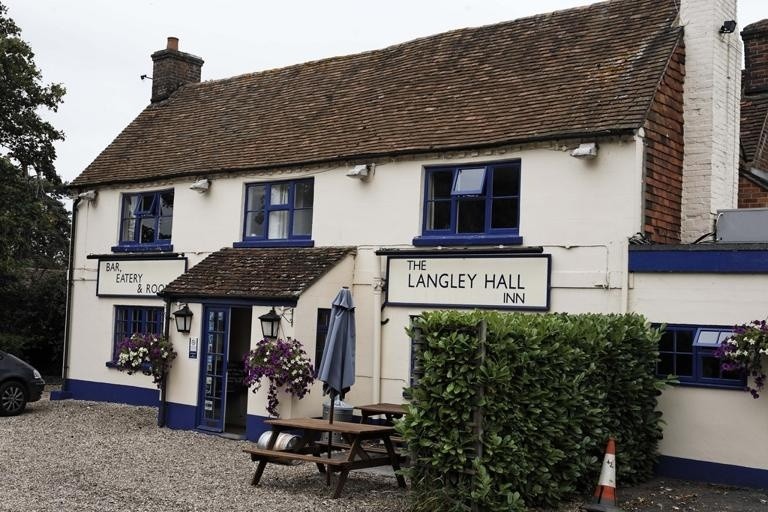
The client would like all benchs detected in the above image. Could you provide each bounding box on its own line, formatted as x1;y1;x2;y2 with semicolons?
241;426;408;499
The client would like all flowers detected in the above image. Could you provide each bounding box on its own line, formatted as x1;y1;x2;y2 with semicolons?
113;331;178;385
712;319;768;401
241;335;317;420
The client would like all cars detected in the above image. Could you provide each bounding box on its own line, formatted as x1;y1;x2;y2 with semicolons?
0;349;45;417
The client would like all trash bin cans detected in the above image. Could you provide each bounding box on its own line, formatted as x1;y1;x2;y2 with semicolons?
320;402;353;444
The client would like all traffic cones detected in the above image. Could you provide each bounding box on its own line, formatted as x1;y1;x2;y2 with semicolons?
580;436;624;510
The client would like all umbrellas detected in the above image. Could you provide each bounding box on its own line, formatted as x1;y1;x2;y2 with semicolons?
316;287;355;486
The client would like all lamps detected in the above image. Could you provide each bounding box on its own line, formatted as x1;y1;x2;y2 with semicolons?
345;163;373;182
569;142;601;161
78;189;97;202
188;175;212;193
257;305;281;340
172;302;193;333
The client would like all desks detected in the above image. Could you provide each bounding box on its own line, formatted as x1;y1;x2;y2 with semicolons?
242;417;407;498
353;402;409;425
